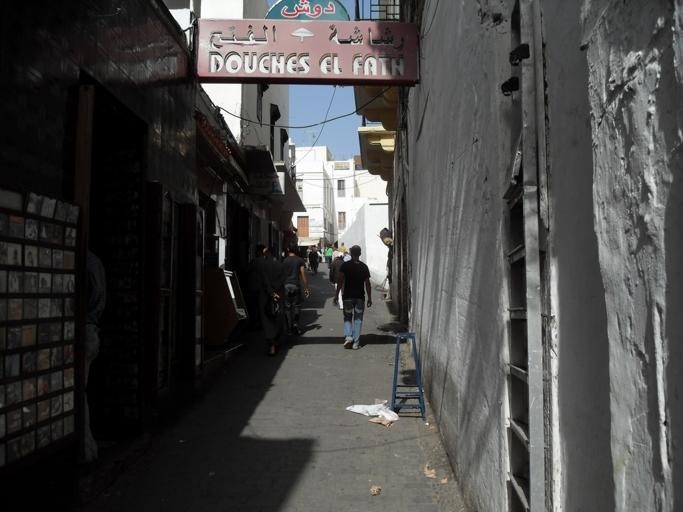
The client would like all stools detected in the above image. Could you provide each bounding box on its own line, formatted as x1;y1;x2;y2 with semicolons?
391;329;425;419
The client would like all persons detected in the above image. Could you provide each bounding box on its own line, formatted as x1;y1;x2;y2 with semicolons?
248;245;288;357
304;246;320;274
324;242;372;350
282;243;313;335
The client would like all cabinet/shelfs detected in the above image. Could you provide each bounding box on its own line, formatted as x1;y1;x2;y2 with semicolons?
0;187;80;466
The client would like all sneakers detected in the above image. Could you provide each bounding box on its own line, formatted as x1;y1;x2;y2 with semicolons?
287;320;301;335
343;340;359;350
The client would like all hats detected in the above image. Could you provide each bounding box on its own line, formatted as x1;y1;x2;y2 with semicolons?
350;245;361;256
